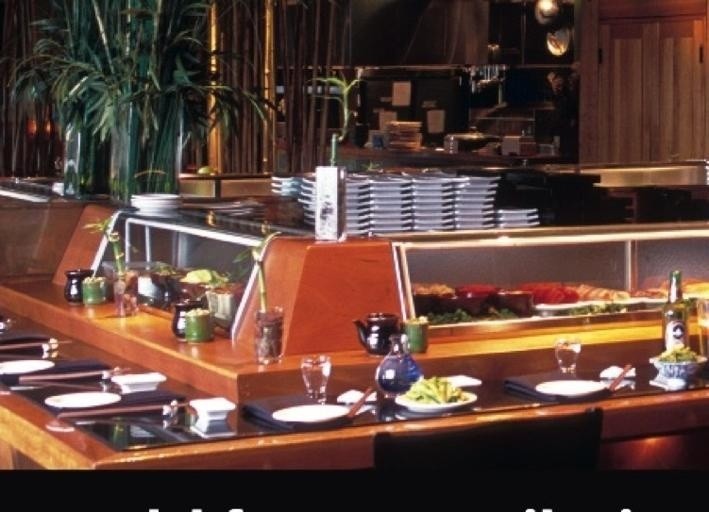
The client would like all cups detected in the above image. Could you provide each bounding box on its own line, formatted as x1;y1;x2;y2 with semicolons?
253;304;284;364
62;267;108;306
401;320;429;355
554;340;582;374
110;268;142;318
300;355;332;402
170;300;214;344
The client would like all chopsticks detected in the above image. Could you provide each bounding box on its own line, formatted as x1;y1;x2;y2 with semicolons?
57;401;163;418
0;336;74;354
17;367;111;385
343;385;375;422
607;362;633;394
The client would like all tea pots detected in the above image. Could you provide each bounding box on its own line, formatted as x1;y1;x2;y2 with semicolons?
352;312;399;358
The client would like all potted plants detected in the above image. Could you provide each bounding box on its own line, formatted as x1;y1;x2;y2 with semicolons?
83;217;141;318
230;230;294;365
303;66;369;242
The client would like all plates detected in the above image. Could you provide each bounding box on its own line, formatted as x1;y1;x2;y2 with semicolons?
188;396;237;423
271;404;350;425
393;391;477;414
43;391;123;411
385;118;426;153
534;379;604;399
129;191;184;213
0;358;56;378
269;172;541;237
109;372;167;396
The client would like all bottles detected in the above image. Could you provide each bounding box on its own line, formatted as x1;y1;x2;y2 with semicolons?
661;268;690;352
374;333;423;400
441;126;460;156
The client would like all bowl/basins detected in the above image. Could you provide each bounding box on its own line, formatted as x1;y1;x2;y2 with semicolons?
648;355;708;381
418;290;535;318
